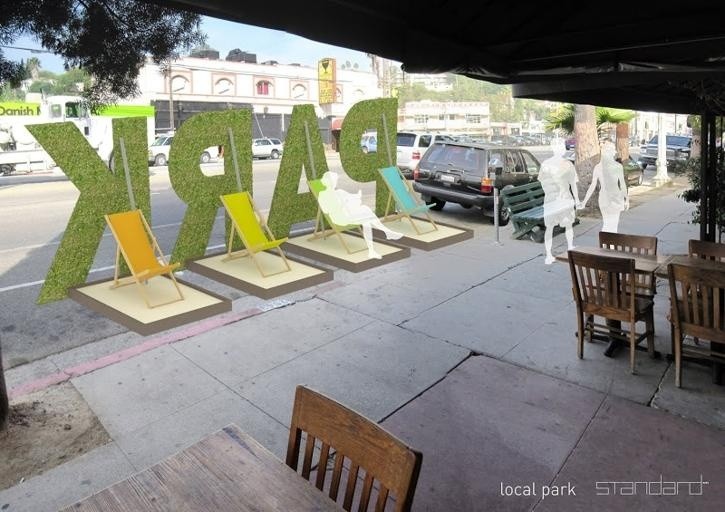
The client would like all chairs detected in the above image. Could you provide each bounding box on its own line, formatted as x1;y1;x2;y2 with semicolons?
568;231;725;389
284;385;423;512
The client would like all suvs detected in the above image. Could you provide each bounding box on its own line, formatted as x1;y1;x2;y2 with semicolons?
395;131;459;177
251;138;284;159
639;134;693;172
412;141;543;226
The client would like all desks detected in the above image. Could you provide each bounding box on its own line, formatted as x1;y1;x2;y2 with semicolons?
58;422;349;512
555;245;725;385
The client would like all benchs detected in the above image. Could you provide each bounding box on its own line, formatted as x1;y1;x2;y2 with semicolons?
500;181;581;244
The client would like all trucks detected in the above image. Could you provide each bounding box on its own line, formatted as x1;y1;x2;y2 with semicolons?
0;89;155;176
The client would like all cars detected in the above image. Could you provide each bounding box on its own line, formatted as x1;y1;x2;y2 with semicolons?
146;134;217;165
493;133;550;146
360;133;377;153
563;149;644;187
565;131;576;149
